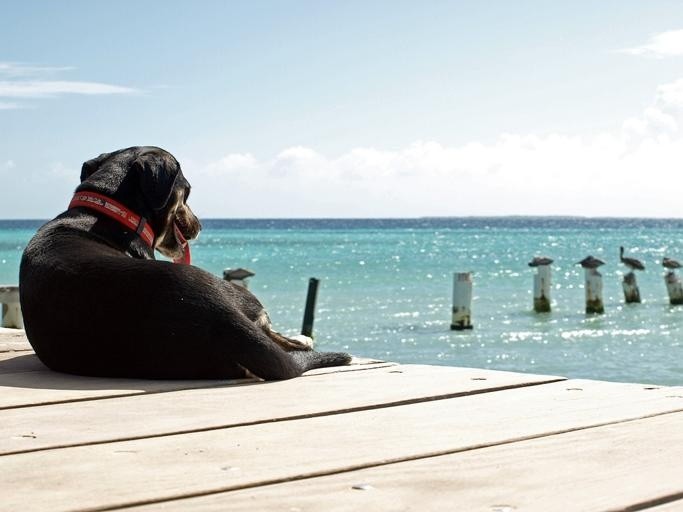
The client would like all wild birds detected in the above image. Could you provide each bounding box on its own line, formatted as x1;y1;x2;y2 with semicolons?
528;256;552;267
661;256;683;275
619;245;644;275
573;255;605;270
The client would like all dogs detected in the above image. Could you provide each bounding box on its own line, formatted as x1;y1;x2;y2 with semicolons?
17;145;354;384
